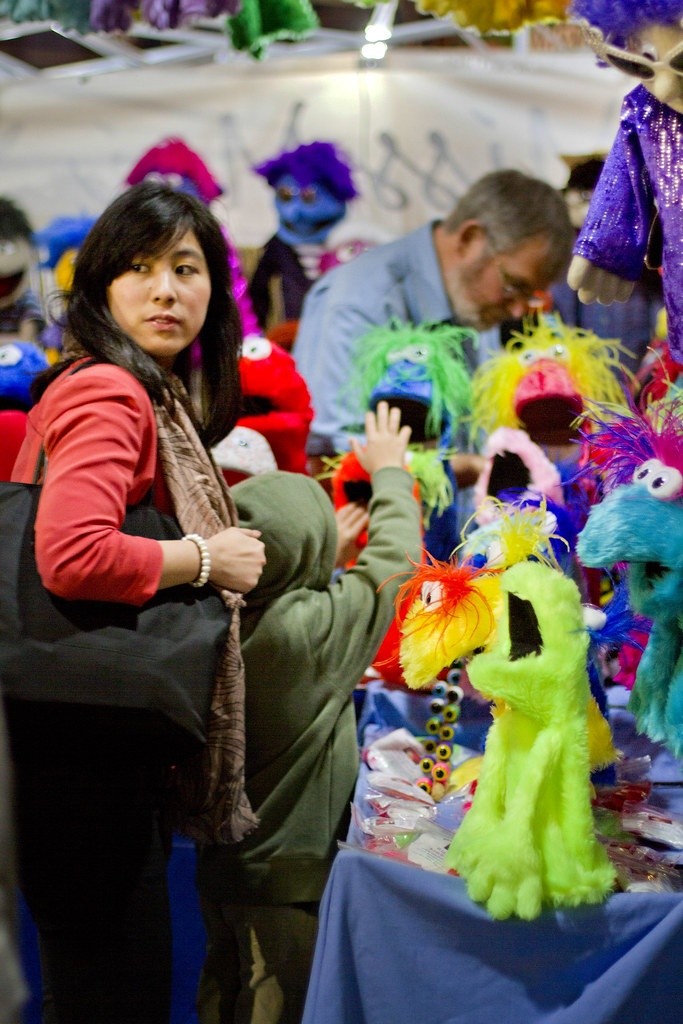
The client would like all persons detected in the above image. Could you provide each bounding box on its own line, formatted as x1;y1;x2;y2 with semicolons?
246;142;356;334
564;0;683;361
552;157;663;382
195;401;423;1024
291;168;577;469
11;182;267;1024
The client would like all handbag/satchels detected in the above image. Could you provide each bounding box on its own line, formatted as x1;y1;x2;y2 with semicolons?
0;359;236;816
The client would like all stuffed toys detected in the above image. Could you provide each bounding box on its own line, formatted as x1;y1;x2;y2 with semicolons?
335;321;683;920
0;139;314;485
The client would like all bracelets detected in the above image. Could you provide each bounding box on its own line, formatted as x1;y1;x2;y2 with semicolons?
181;534;211;588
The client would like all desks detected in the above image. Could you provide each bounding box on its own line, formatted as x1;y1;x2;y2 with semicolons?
298;678;680;1024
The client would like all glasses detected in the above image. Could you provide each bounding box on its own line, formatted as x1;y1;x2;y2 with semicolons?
484;237;543;312
584;39;683;80
